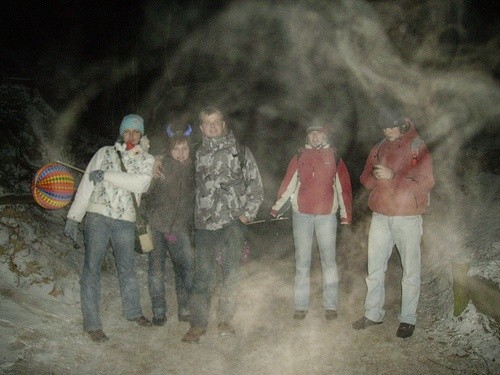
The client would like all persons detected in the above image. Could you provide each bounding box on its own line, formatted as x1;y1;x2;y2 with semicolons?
353;103;435;337
270;116;352;319
63;114;156;342
135;131;195;326
151;105;265;343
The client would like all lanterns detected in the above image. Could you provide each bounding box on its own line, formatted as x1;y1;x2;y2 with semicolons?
31;161;76;210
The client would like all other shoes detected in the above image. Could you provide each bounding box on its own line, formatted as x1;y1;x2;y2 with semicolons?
325;310;338;320
218;321;235;336
152;314;167;326
352;317;383;330
181;327;206;343
87;329;109;343
293;310;308;320
127;316;152;327
396;323;415;338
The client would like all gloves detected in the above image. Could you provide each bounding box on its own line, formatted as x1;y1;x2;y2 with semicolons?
64;218;79;241
89;170;104;186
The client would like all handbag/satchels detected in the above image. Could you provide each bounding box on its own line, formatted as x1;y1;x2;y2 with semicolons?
135;217;156;253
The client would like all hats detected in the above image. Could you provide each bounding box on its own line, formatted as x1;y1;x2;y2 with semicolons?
119;114;144;137
378;105;405;129
306;119;329;134
